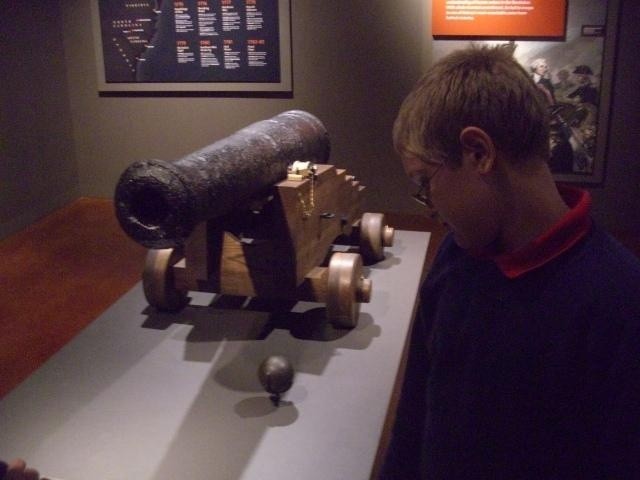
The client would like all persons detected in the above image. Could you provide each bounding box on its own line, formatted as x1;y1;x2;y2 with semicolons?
375;41;640;479
546;64;598;168
529;58;550;85
0;457;51;480
551;69;576;96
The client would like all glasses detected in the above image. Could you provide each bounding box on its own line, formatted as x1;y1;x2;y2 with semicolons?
411;155;450;207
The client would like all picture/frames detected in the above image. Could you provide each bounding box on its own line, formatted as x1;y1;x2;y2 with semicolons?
420;0;620;184
90;0;292;93
